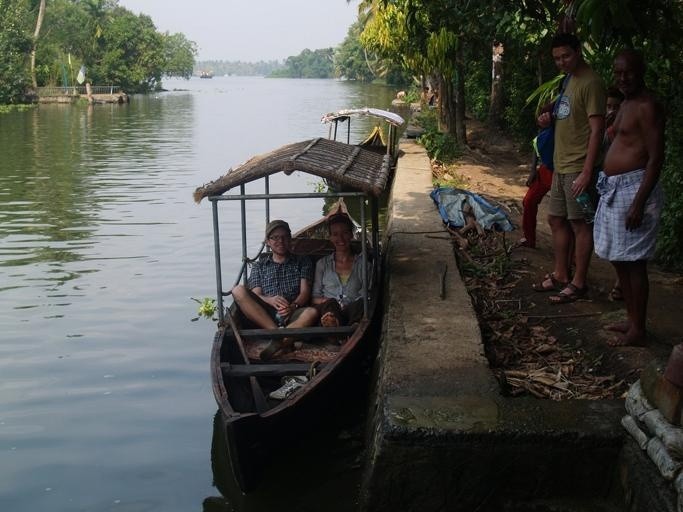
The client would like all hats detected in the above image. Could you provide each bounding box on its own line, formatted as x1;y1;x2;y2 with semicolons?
264;220;291;237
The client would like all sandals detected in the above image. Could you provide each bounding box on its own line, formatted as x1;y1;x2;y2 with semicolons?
610;281;623;301
548;283;588;304
532;273;569;291
512;238;537;248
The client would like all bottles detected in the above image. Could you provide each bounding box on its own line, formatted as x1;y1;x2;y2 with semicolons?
575;193;596;224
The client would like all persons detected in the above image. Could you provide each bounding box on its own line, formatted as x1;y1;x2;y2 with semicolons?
516;164;553;250
231;218;318;347
601;88;625;303
591;48;666;347
308;211;374;326
529;32;607;306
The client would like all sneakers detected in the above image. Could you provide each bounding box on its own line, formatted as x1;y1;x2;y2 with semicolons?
259;326;304;362
269;375;309;400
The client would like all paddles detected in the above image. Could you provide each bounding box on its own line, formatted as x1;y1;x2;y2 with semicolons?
225;307;266;414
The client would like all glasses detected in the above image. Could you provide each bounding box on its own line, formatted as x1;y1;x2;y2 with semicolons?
328;213;348;234
268;234;292;241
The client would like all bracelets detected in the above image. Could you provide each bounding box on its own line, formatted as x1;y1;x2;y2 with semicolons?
292;300;301;308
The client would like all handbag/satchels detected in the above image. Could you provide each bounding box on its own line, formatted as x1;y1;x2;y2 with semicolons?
538;127;553;170
